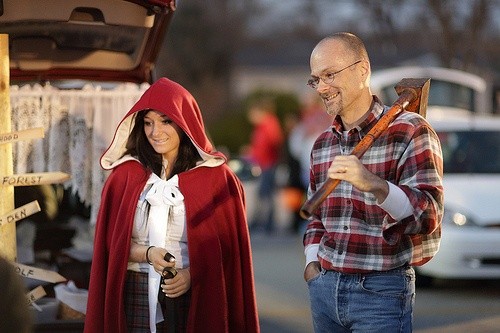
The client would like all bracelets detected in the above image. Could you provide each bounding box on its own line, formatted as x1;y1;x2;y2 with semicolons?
146;246;155;265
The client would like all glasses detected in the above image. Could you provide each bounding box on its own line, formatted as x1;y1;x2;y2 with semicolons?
307;60;362;87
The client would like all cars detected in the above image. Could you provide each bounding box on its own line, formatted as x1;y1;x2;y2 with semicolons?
414;105;500;288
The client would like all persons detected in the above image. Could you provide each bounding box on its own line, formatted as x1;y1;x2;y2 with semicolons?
302;31;445;333
244;101;283;237
83;77;260;333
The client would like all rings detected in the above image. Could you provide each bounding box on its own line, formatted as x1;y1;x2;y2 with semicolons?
344;165;348;172
